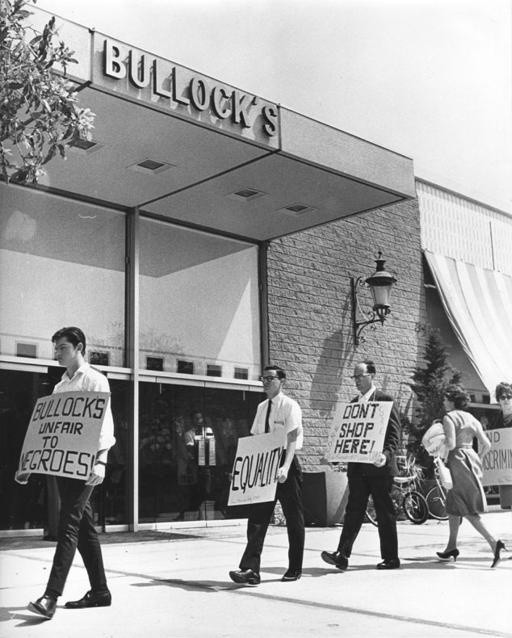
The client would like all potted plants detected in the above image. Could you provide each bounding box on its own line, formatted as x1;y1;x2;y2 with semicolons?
400;327;473;520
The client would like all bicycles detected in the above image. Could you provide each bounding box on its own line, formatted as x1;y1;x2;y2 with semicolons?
426;464;463;525
361;461;432;528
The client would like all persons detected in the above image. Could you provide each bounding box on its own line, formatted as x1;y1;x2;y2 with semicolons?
183;405;218;516
321;359;401;570
17;325;117;620
477;381;512;433
229;364;305;584
422;385;509;566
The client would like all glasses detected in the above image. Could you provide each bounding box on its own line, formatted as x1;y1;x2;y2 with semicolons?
351;373;370;379
259;376;280;382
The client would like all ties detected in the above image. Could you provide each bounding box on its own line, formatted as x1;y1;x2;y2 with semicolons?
265;400;272;433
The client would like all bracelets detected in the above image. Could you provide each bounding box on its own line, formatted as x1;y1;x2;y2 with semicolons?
94;458;109;466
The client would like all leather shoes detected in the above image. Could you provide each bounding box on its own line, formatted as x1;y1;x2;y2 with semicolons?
229;568;260;584
282;569;302;581
321;551;349;570
66;591;111;609
27;594;57;620
377;559;400;569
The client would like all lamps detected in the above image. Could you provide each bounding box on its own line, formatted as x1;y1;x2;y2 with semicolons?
347;248;399;348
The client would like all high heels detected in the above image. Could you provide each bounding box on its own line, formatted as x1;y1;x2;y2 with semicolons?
490;540;508;568
436;549;459;561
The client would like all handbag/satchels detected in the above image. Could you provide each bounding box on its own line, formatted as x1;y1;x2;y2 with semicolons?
434;457;453;490
